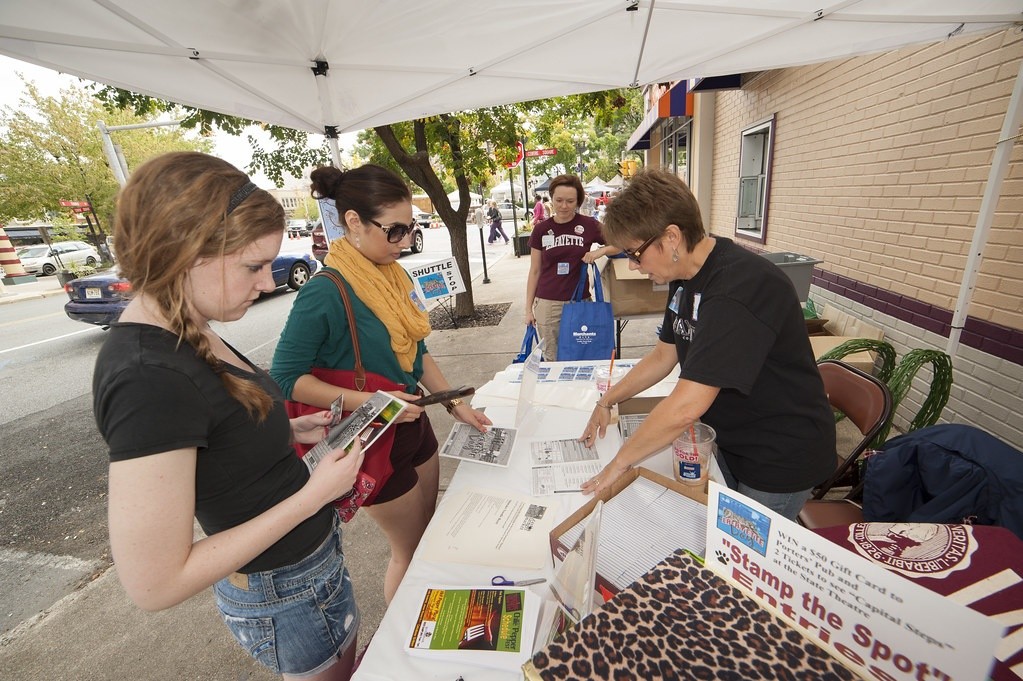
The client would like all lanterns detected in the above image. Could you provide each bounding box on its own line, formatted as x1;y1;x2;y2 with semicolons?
534;177;554;195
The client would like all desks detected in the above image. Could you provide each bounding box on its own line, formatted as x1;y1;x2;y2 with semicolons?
611;311;665;359
344;356;729;681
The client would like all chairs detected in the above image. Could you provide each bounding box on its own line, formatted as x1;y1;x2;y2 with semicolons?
796;424;1023;543
806;359;892;503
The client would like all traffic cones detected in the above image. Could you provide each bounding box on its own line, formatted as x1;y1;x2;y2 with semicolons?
296;231;301;239
290;231;294;240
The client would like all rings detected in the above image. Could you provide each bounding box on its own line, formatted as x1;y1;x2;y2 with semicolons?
595;479;599;485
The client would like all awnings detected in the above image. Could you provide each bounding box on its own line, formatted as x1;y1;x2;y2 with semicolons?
686;71;763;94
626;80;694;152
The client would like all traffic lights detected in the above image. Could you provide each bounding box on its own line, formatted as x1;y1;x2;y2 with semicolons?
617;160;628;176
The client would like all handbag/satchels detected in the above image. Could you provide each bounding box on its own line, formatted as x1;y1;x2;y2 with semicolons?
512;326;545;363
492;208;501;222
284;269;408;508
557;261;617;361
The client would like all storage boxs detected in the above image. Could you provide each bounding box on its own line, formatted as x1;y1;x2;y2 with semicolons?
549;466;709;607
588;245;671;317
804;303;885;376
616;396;703;447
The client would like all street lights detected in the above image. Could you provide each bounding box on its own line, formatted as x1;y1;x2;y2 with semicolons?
574;133;587;181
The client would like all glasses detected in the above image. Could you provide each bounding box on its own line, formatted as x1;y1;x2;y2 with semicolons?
623;222;685;264
352;210;417;243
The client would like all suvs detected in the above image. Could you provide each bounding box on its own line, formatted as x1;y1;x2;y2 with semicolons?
288;219;308;237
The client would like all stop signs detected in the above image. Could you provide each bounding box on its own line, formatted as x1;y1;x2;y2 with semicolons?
494;140;523;168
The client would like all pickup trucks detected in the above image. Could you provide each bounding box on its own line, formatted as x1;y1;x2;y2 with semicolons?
587;190;616;205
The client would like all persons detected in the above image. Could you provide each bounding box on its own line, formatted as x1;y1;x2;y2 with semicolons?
93;151;366;681
270;164;493;603
578;167;837;523
530;195;544;225
542;196;552;217
486;200;502;241
488;200;509;243
580;193;607;223
525;175;624;361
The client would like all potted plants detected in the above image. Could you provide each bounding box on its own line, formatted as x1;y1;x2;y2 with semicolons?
51;258;98;288
511;220;534;256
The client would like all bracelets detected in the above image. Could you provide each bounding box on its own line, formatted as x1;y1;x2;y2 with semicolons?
447;398;462;415
596;400;613;409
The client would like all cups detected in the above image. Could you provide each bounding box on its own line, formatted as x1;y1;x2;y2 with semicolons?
672;422;716;487
595;364;626;425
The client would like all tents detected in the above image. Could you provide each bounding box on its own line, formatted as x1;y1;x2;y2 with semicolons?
490;182;522;199
583;176;615;192
447;189;482;206
0;0;1023;363
605;174;630;187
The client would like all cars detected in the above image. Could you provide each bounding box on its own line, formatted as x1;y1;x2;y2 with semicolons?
15;241;102;277
312;209;424;266
412;205;431;228
482;203;534;221
63;252;317;330
